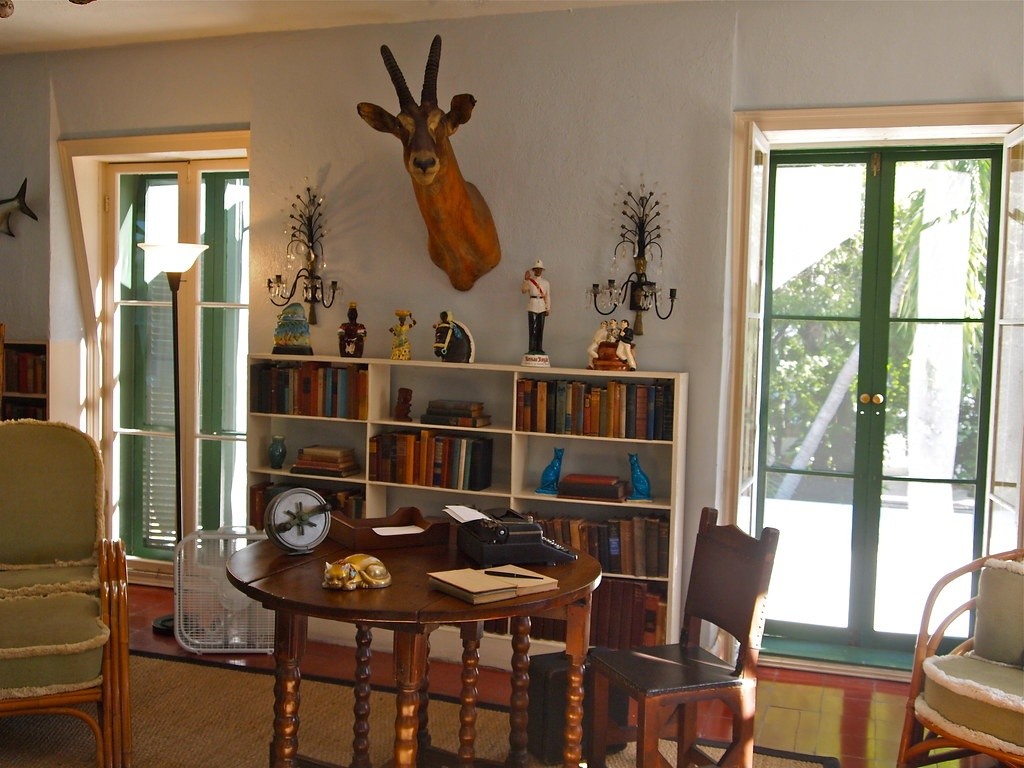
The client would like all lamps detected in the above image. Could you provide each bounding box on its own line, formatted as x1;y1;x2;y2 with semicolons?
137;242;210;635
264;176;345;325
587;171;676;335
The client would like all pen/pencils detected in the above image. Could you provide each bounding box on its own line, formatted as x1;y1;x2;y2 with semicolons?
485;571;545;580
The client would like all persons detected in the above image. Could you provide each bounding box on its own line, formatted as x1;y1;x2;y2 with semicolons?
337;299;367;358
522;259;551;356
387;310;417;360
586;317;636;371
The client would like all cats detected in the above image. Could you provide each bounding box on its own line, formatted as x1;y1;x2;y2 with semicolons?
322;554;392;591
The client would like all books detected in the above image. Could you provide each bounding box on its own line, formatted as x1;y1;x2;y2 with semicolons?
3;351;46;393
2;401;46;420
484;580;666;652
289;443;361;477
369;399;494;491
249;364;368;421
534;516;669;579
514;377;673;442
425;563;560;605
250;482;366;532
559;474;632;504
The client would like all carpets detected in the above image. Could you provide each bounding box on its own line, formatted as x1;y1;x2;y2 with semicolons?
1;649;841;768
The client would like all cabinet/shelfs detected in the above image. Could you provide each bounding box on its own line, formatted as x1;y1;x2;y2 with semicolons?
0;338;49;422
245;353;689;709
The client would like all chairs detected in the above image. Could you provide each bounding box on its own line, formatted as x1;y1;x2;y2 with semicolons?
1;419;131;768
896;547;1024;768
588;507;780;768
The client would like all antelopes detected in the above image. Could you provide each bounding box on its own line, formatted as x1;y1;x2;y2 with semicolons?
357;36;501;291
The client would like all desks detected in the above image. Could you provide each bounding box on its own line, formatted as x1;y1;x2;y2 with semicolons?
224;521;603;768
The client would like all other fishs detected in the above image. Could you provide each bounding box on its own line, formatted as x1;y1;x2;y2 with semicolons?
0;177;39;237
